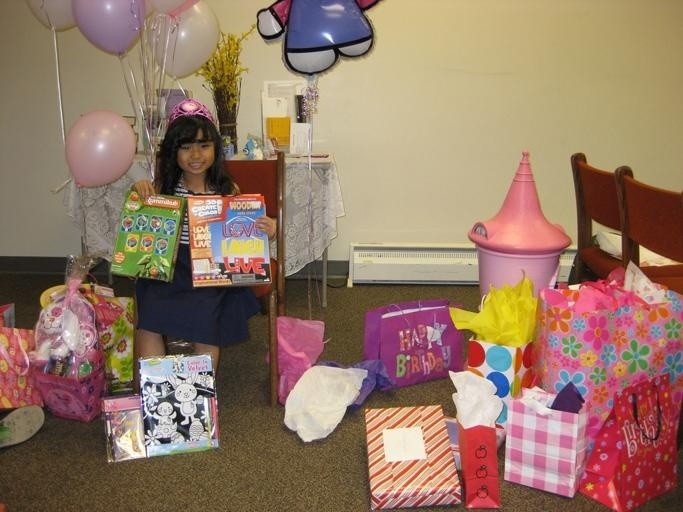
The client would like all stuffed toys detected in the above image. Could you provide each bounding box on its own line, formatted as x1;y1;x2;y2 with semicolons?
27;304;81;361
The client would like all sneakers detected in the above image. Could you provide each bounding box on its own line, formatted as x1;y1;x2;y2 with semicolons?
0;405;46;450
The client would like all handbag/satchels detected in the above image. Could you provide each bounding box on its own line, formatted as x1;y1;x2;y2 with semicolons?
0;303;46;408
363;299;464;393
456;276;682;511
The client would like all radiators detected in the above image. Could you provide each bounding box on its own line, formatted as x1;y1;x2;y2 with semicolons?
347;242;577;288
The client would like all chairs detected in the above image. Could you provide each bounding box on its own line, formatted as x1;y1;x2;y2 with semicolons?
566;152;631;287
612;167;682;299
221;152;287;407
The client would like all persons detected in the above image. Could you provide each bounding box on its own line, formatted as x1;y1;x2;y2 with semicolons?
133;100;278;380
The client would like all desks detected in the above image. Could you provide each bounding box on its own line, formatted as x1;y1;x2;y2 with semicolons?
64;150;345;350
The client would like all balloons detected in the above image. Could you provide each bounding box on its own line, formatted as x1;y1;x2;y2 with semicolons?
29;1;220;189
256;0;379;78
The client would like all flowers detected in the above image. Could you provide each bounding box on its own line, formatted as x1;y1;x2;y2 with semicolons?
192;25;258;155
